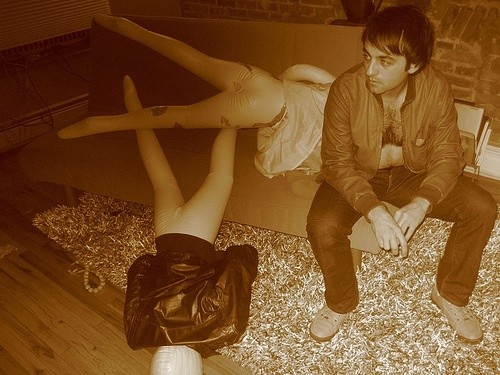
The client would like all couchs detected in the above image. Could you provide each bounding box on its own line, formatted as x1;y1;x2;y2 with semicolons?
18;13;399;270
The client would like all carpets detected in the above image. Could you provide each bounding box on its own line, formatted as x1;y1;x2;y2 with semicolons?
33;191;499;374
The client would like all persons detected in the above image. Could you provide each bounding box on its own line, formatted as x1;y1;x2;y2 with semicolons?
306;6;498;343
57;11;337;179
123;75;260;375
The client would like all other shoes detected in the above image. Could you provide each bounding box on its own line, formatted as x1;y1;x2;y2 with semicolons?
429;291;484;344
308;306;350;342
